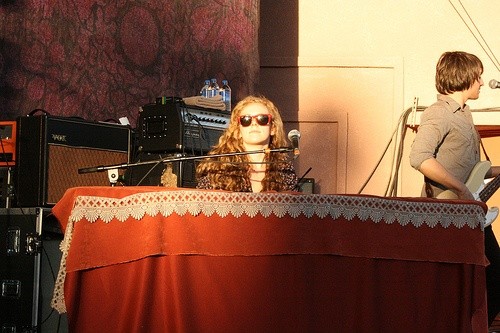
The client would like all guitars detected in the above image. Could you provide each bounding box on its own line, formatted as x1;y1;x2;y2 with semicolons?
436;160;500;228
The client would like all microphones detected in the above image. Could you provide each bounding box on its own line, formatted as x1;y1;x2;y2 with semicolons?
288;129;301;154
489;79;500;89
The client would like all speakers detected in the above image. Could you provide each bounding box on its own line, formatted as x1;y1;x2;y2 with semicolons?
135;152;203;186
0;167;16;209
15;116;130;207
298;178;314;194
0;209;68;333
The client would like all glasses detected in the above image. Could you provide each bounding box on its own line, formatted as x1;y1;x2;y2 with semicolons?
237;114;272;128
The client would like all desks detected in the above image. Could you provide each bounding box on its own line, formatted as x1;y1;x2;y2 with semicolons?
49;186;490;333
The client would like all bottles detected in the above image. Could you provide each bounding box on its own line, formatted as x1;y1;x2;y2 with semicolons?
200;80;211;98
220;80;231;111
207;79;220;98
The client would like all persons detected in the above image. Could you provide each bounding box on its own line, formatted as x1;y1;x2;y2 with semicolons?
410;51;500;328
194;95;301;190
